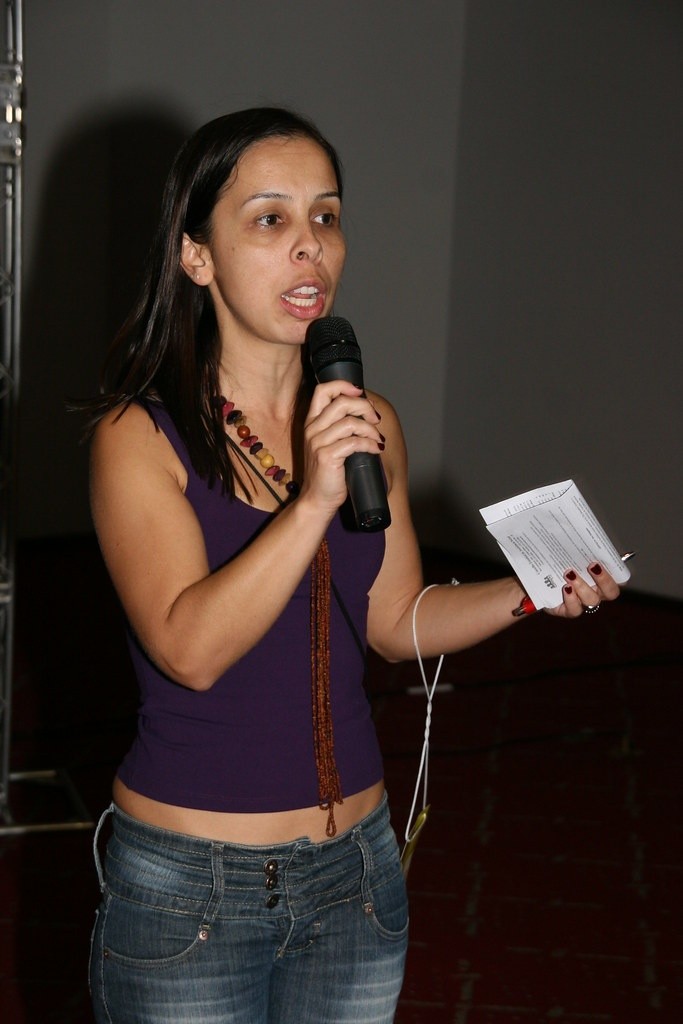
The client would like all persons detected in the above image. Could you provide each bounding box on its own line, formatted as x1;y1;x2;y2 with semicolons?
86;107;622;1024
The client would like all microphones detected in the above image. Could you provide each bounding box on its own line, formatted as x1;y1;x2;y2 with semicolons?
306;316;392;533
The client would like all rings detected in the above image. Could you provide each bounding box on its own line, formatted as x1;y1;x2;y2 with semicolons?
583;604;600;614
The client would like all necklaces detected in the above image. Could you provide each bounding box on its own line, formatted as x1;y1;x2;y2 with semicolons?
221;394;298;496
